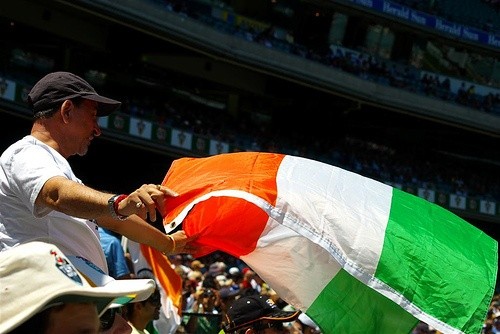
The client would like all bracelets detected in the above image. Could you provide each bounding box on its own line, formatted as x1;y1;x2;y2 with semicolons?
161;235;176;257
108;193;129;223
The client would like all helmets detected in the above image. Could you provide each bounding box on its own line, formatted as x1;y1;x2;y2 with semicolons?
0;241;157;334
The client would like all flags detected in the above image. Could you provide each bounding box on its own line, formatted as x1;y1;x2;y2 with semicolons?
157;151;498;334
121;236;182;334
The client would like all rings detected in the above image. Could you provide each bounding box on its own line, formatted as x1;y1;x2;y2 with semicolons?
136;202;142;208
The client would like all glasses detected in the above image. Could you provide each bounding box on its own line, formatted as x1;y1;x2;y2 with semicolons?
256;320;284;332
149;295;160;307
100;307;121;331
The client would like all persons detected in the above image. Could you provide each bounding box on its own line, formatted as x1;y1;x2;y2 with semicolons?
0;72;188;273
0;242;321;334
119;0;500;200
413;295;500;334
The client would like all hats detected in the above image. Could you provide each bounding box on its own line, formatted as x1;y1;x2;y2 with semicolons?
189;271;202;280
203;261;240;289
186;254;196;260
28;71;122;118
222;294;300;332
191;260;205;270
261;283;322;332
